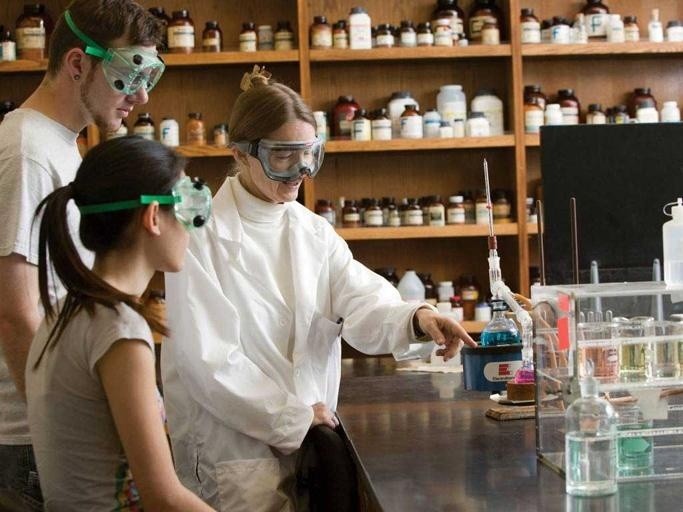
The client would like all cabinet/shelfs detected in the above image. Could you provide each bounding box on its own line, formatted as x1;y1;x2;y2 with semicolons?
0;0;683;362
522;269;683;486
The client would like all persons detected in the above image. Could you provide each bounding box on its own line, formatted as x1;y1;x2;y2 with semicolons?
25;135;217;512
0;0;167;512
161;64;479;512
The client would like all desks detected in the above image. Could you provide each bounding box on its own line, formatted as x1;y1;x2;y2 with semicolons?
327;354;682;511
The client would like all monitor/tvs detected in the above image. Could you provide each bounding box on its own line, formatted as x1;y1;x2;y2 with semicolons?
540;122;683;285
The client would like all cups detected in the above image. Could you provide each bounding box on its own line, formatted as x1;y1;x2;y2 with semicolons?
575;311;683;384
617;408;657;470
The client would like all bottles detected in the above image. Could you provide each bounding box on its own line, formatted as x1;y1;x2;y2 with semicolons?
145;5;294;50
103;109;229;148
316;190;509;227
369;268;492;322
519;0;683;45
429;372;463;400
0;2;50;62
314;84;504;142
561;374;620;500
337;407;475;438
523;82;682;133
516;328;537;382
657;196;683;284
481;296;521;345
309;0;503;50
